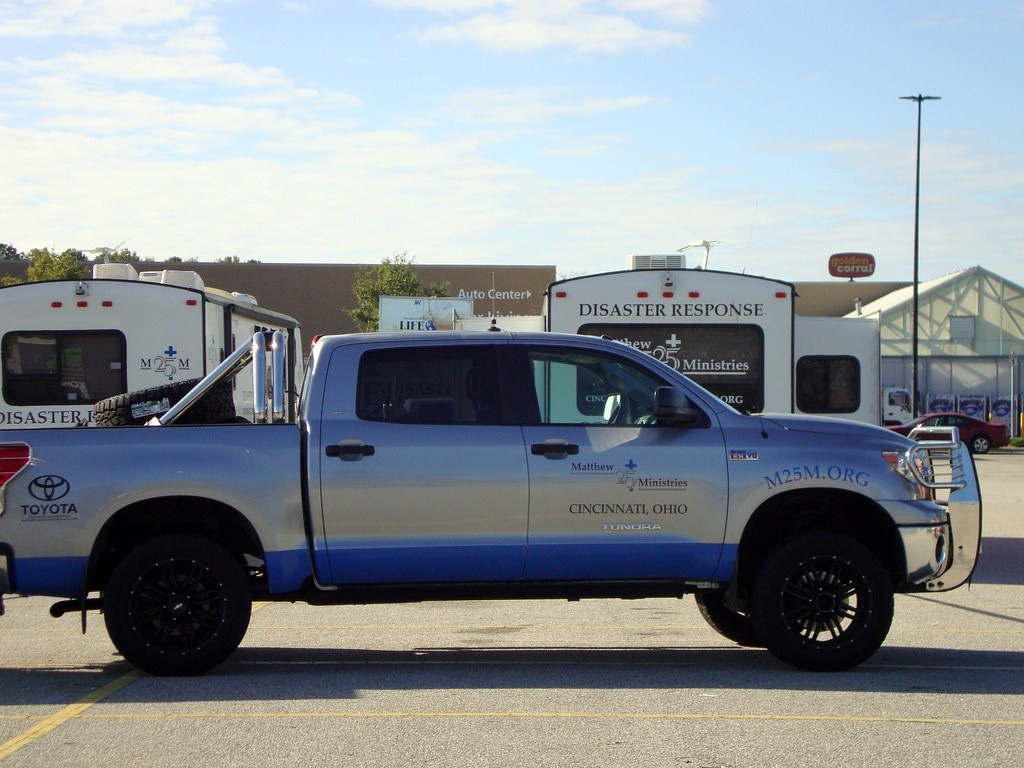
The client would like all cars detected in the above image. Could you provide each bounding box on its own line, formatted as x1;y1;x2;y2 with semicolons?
882;412;1011;454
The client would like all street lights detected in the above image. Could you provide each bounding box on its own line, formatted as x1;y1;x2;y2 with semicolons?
899;94;943;421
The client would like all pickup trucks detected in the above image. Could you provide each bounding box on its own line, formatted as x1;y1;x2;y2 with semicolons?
0;328;986;676
